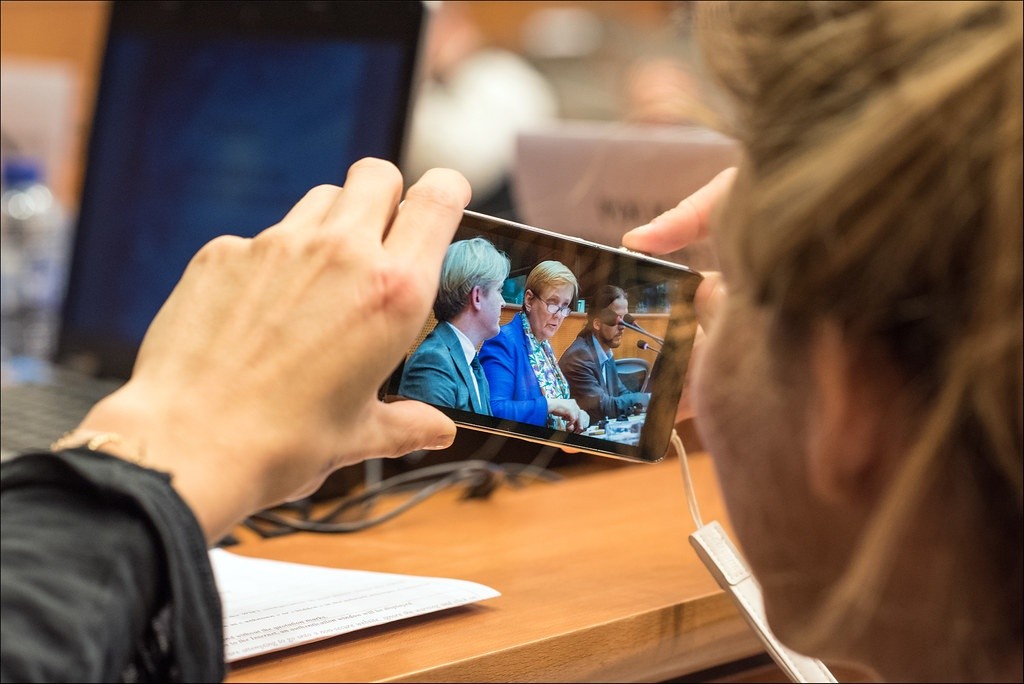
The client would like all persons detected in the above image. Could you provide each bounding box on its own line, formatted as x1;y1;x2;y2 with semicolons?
398;237;512;418
1;2;1023;684
477;261;591;436
558;285;653;428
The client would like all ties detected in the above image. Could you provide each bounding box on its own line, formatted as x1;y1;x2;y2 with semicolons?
470;353;494;417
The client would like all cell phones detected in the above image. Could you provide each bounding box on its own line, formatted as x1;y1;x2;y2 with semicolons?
377;200;704;465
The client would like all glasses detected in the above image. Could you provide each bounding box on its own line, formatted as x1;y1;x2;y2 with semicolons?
533;293;572;317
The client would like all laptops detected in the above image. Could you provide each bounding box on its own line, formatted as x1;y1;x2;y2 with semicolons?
1;0;434;465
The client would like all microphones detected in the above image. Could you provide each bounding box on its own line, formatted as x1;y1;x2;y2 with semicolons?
600;308;665;353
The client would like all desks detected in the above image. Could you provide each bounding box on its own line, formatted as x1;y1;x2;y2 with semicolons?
221;419;789;684
403;305;670;375
